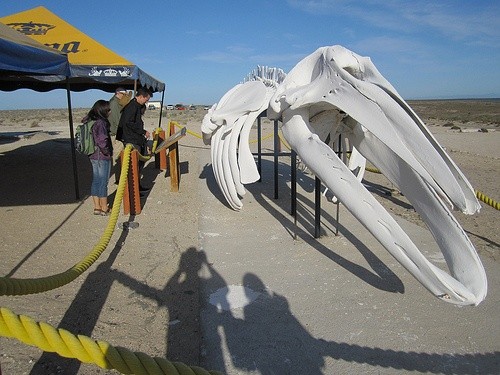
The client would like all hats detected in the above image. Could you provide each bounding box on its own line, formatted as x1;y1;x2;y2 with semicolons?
116;88;127;94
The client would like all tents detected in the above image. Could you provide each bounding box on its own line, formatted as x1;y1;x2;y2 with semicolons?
0;5;166;128
0;22;79;201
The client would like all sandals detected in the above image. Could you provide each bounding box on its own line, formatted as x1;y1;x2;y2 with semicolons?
94;208;112;216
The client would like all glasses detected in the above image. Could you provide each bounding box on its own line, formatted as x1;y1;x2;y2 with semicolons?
119;92;127;96
108;108;111;112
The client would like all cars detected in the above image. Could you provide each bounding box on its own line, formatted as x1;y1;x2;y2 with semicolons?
167;104;174;110
148;104;156;110
189;105;197;110
175;104;187;110
203;105;209;110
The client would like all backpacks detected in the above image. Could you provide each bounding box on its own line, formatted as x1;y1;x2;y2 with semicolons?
75;120;100;156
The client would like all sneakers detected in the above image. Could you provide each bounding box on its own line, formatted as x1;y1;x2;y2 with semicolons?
139;188;150;199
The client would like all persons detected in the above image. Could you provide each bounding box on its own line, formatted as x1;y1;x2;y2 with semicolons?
81;100;112;216
107;88;127;184
115;88;153;198
